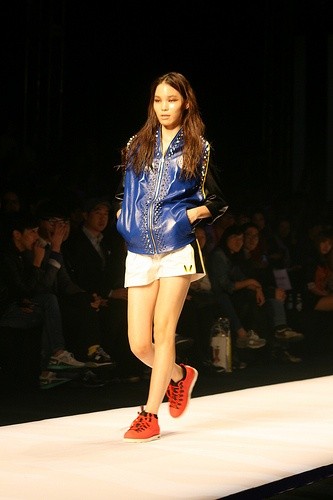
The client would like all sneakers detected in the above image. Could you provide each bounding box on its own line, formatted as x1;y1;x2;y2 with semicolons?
236;329;266;349
121;405;160;443
38;371;71;391
86;345;112;367
273;327;304;344
46;350;86;371
165;363;198;419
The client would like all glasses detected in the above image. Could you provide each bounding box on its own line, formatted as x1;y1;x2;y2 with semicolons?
38;218;66;226
243;231;260;240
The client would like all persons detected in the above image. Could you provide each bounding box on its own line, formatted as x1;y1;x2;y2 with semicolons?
116;72;229;445
0;191;333;391
